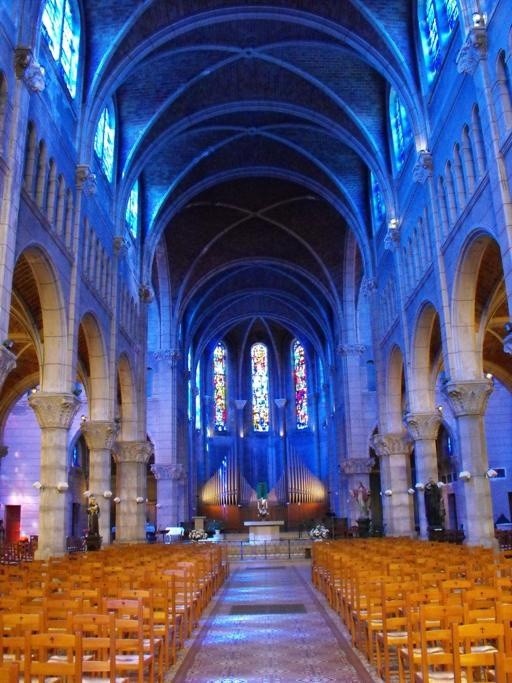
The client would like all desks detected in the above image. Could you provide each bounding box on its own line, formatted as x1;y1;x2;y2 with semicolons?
244;520;285;544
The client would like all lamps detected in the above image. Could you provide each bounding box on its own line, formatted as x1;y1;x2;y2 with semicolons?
34;481;146;505
377;469;498;496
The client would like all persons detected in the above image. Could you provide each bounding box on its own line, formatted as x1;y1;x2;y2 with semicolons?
87;497;100;539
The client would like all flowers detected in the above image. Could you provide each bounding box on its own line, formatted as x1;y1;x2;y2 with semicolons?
307;525;331;542
189;528;209;541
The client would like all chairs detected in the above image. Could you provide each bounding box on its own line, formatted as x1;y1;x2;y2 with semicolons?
0;541;226;682
309;536;512;683
